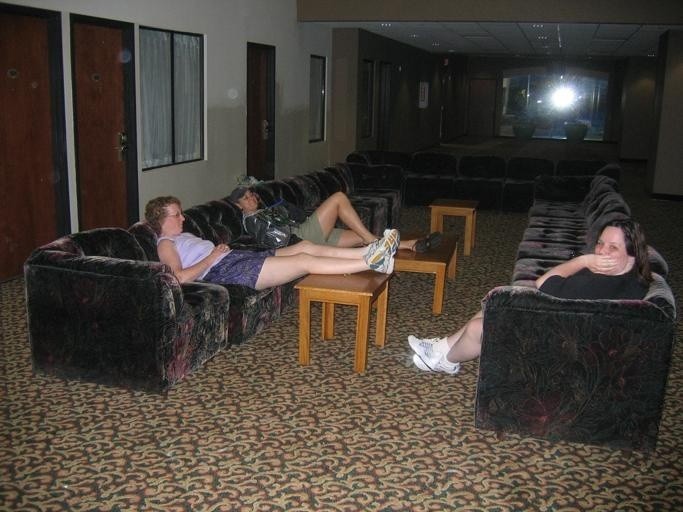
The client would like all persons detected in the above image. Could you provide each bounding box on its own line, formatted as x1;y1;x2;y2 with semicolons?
228;187;450;253
406;215;654;375
141;195;402;289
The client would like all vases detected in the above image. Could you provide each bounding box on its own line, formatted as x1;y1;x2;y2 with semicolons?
564;120;591;140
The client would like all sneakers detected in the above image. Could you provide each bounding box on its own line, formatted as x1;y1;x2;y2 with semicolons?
367;228;404;277
410;228;446;256
407;329;441;370
411;353;460;379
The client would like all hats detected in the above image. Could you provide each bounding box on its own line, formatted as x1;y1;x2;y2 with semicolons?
229;185;252;200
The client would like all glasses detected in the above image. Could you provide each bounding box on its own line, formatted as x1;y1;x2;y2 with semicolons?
166;208;181;219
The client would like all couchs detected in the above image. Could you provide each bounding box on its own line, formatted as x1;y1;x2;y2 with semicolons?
475;174;677;453
23;162;402;395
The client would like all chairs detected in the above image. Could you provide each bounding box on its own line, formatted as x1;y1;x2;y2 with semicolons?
347;150;621;210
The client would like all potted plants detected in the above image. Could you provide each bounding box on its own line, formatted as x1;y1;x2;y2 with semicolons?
512;87;536;138
